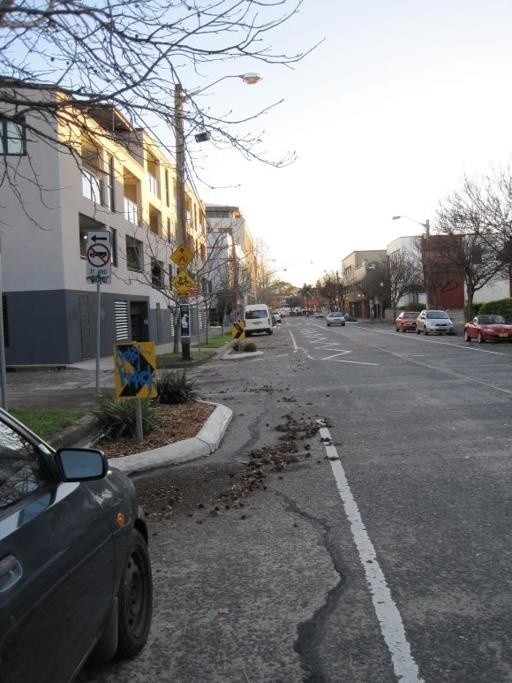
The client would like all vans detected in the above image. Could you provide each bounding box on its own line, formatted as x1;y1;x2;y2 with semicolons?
244;304;274;335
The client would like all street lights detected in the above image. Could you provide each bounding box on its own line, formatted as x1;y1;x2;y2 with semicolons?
176;73;263;361
392;215;430;310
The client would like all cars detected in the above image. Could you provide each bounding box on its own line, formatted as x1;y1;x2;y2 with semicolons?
0;405;152;681
396;312;420;332
272;311;282;323
415;309;454;336
326;311;346;327
464;315;512;343
